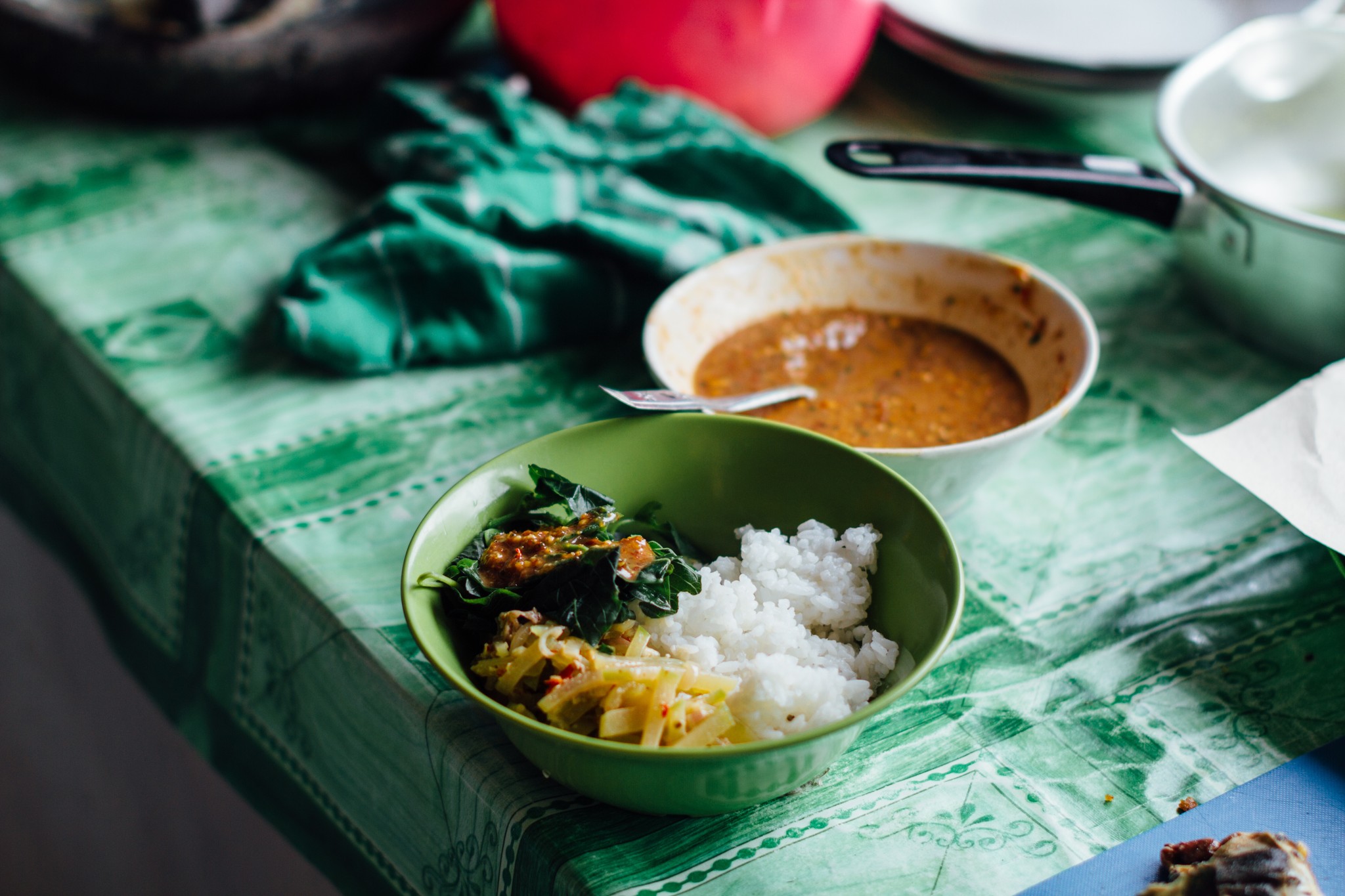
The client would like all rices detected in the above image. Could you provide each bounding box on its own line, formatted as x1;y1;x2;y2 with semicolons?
620;503;899;736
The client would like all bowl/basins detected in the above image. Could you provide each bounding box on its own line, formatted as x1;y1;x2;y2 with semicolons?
644;235;1100;507
403;414;966;820
878;0;1316;96
490;0;883;145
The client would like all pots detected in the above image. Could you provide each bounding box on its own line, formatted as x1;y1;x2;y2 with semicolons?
825;0;1344;372
